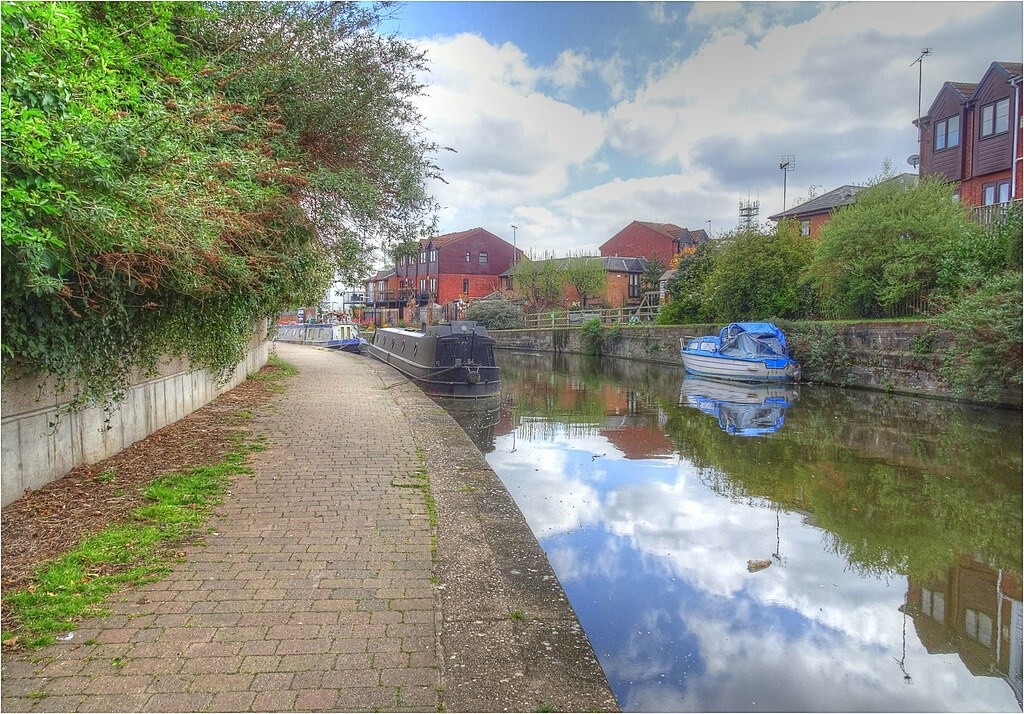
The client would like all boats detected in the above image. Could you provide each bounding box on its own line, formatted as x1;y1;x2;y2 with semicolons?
679;375;801;437
680;322;801;382
368;321;501;398
272;323;369;355
